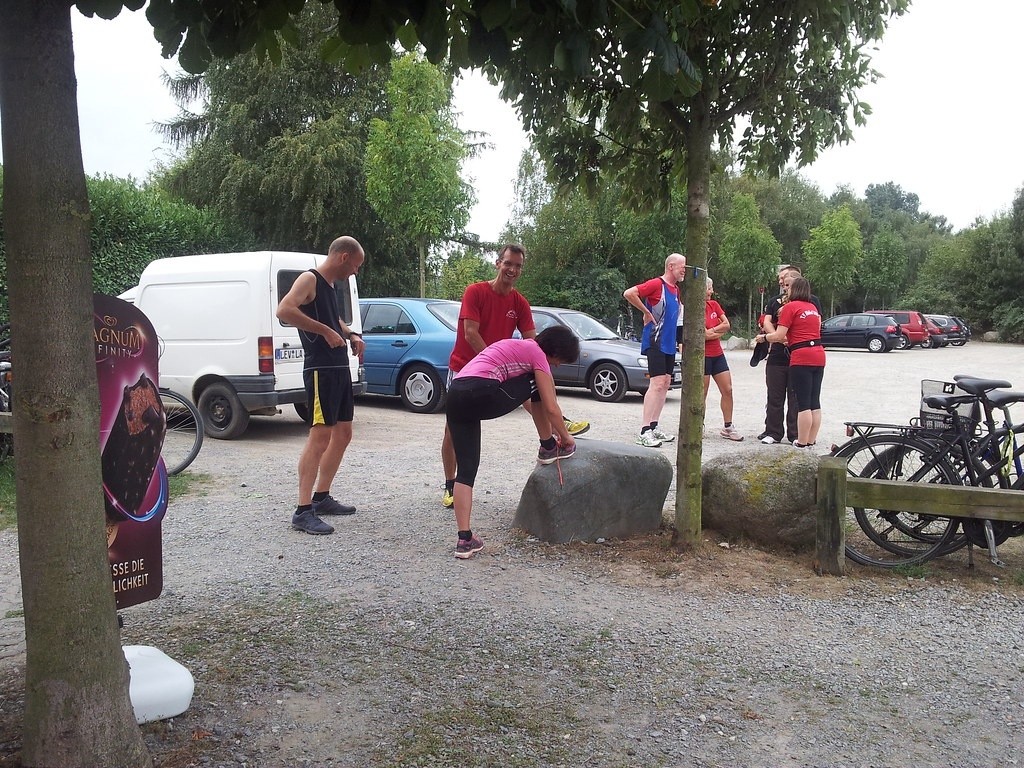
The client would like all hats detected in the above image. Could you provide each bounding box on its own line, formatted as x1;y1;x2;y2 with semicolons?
749;341;769;368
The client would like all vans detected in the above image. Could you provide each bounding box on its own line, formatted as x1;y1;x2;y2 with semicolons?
115;249;368;441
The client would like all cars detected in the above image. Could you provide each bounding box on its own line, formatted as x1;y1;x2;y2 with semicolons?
919;314;972;350
512;305;683;404
356;297;462;414
820;312;902;353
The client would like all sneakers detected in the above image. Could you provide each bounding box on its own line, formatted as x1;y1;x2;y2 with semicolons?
701;423;707;439
652;425;675;442
454;531;484;559
635;429;662;447
291;510;335;535
536;439;577;487
441;483;454;508
310;494;356;515
718;424;744;441
550;415;590;436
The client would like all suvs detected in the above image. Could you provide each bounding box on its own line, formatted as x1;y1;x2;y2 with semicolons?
837;309;932;351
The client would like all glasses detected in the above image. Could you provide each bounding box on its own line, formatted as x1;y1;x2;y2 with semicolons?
497;257;524;270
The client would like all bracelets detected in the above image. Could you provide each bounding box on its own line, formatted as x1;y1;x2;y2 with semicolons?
348;332;359;340
764;335;768;342
711;327;716;333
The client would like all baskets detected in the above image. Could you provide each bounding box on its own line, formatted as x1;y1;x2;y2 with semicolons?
919;378;982;437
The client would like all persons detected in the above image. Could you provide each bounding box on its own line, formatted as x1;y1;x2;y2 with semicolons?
277;234;365;536
702;277;744;446
444;324;580;559
440;242;591;507
756;266;826;449
624;253;686;448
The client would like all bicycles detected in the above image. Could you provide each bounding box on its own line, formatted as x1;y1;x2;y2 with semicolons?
828;373;1024;570
0;324;205;478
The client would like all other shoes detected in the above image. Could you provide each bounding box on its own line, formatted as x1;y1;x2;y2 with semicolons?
757;430;768;439
761;434;781;444
792;439;814;453
